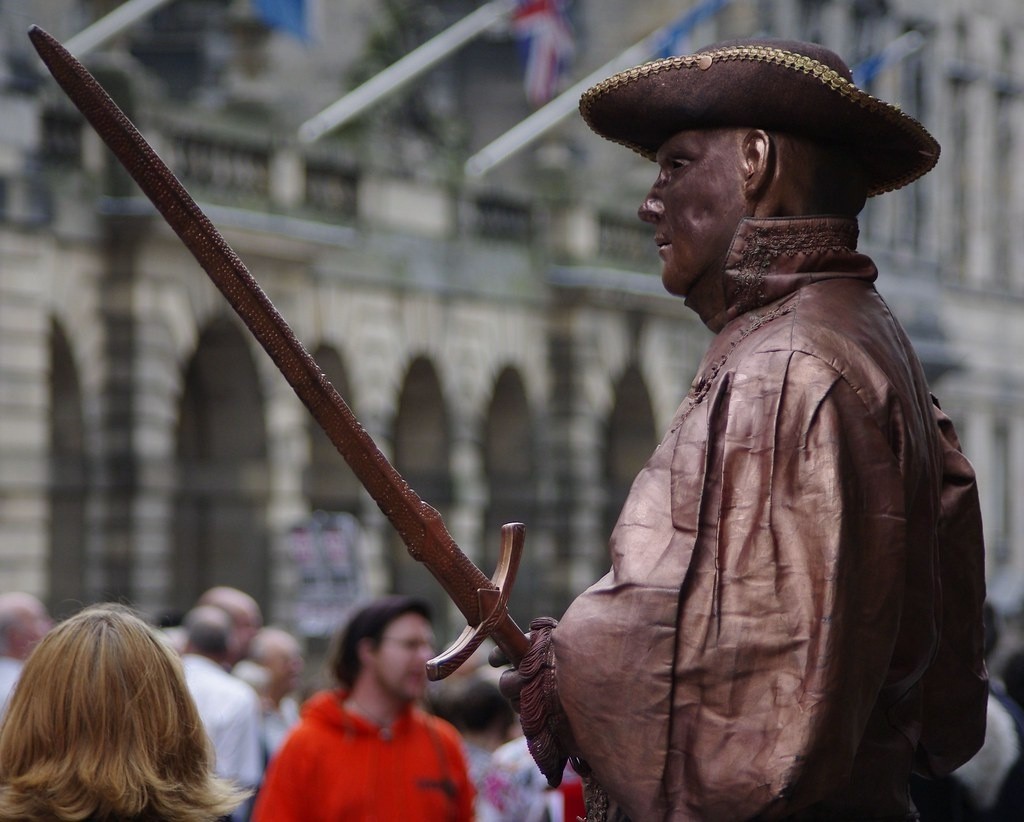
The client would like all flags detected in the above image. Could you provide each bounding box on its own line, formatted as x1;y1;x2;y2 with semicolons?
247;0;308;50
490;0;580;106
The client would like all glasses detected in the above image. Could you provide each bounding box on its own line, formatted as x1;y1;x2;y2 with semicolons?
381;637;438;651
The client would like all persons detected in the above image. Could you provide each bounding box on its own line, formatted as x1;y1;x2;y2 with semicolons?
487;37;991;821
0;585;584;822
